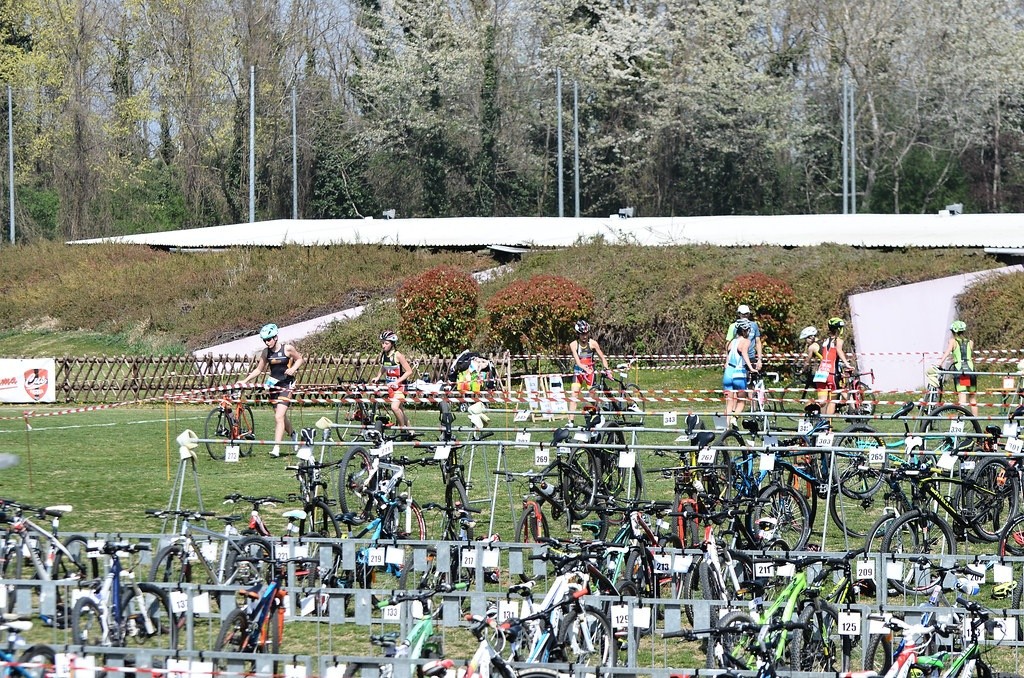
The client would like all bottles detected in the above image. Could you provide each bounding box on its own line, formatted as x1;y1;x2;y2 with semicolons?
228;407;233;420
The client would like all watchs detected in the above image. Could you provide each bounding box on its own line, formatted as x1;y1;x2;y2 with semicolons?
757;357;762;360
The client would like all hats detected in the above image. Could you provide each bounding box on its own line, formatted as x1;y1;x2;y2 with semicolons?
737;305;750;314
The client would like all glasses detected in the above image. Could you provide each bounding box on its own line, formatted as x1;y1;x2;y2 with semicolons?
263;338;271;342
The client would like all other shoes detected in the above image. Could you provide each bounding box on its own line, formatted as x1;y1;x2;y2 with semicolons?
269;446;280;457
564;420;574;428
730;416;739;430
290;430;298;451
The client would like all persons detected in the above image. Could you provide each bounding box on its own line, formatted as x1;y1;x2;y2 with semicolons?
722;305;763;432
564;320;615;428
28;369;39;400
371;331;415;441
236;323;304;456
936;320;978;434
799;317;855;431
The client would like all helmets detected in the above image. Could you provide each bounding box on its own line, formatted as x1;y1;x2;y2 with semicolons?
735;318;751;330
828;318;844;327
991;580;1018;598
800;326;817;339
260;324;278;339
950;321;966;333
575;320;590;333
380;331;398;342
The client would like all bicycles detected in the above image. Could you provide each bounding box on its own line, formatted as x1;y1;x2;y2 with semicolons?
582;363;646;427
222;412;494;618
419;614;563;678
211;556;321;678
0;613;57;678
334;377;399;442
204;389;255;460
918;368;946;431
743;362;876;431
342;581;470;678
145;508;276;610
492;402;1024;678
71;541;177;678
0;498;99;627
